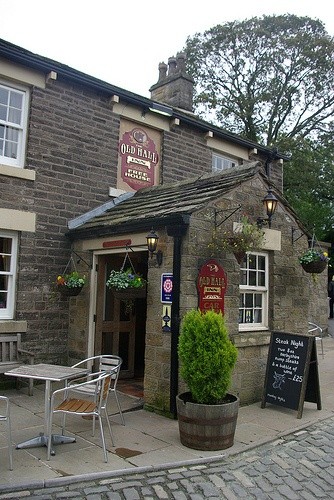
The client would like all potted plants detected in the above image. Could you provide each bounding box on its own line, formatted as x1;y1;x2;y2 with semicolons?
106;246;147;303
207;215;266;267
175;307;240;451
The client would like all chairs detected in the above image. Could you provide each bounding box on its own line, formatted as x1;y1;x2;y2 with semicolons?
46;369;116;463
61;354;127;438
0;395;14;471
307;320;325;358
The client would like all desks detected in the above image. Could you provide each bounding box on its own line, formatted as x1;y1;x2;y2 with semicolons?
4;362;91;457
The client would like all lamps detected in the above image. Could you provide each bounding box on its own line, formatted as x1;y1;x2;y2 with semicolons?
145;226;164;267
256;189;278;229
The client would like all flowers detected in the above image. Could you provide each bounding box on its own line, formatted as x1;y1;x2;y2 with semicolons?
299;247;331;263
56;272;85;288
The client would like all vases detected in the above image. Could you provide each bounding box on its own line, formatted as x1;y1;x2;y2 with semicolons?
302;262;329;274
57;283;84;296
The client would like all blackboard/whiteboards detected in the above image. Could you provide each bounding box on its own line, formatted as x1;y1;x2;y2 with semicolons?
261;331;321;411
